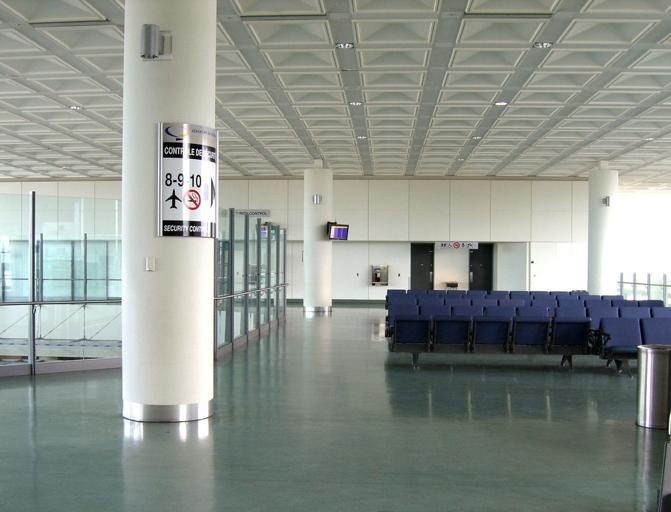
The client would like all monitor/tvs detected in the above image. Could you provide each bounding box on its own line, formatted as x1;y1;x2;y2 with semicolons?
328;223;349;241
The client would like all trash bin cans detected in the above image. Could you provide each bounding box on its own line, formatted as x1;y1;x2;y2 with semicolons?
636;344;671;429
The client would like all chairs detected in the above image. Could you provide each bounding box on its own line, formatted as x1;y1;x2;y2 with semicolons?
384;290;669;378
